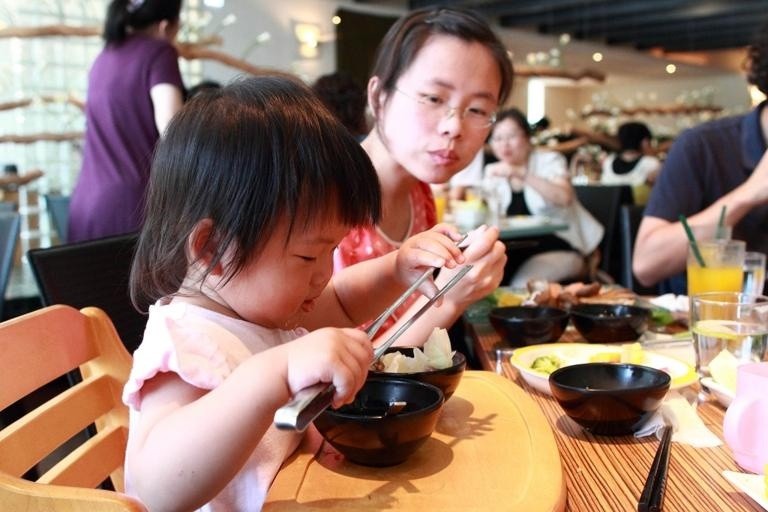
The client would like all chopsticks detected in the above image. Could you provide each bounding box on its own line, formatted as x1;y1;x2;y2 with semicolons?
638;421;673;511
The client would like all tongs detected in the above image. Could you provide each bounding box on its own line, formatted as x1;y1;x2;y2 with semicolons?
270;232;474;431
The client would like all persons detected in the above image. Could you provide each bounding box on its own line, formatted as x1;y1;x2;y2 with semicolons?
598;120;662;186
302;68;368;142
121;68;464;512
630;19;766;300
179;80;225;99
477;106;609;292
65;0;185;247
327;4;511;353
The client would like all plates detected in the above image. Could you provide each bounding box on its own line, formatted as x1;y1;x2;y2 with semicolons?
508;340;698;399
700;376;732;407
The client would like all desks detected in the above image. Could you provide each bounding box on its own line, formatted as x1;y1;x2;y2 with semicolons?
260;369;567;510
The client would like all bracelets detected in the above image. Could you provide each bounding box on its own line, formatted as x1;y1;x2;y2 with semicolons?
520;167;530;181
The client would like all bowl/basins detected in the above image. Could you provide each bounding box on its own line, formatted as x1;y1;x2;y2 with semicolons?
549;361;672;438
487;305;570;345
570;302;654;342
312;377;443;465
364;346;467;401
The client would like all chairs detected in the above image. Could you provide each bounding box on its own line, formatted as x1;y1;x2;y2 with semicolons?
28;230;159;490
47;196;70;241
1;192;22;299
491;183;689;295
0;304;151;511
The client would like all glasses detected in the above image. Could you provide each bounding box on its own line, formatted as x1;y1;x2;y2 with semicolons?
394;86;497;129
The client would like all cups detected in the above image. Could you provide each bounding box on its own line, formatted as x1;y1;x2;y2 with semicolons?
721;362;767;474
688;291;768;401
744;251;766;296
681;224;733;243
455;209;485;231
685;241;745;301
